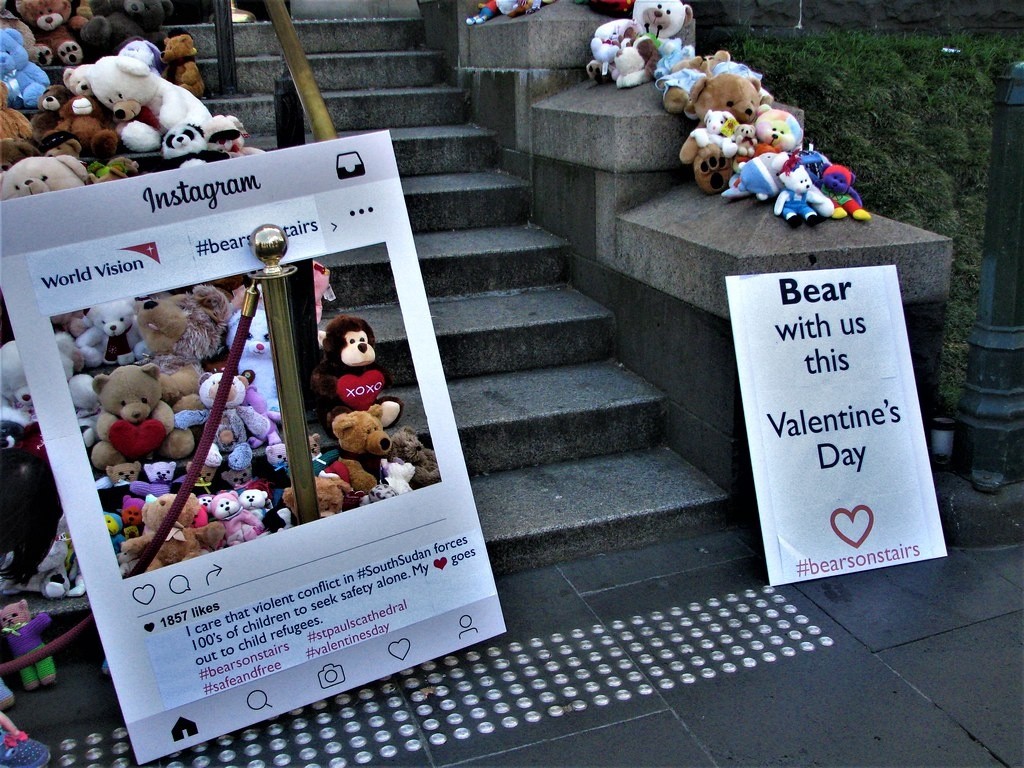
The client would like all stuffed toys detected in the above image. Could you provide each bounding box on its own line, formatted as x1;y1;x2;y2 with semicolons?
462;0;563;26
584;0;874;226
0;0;445;768
